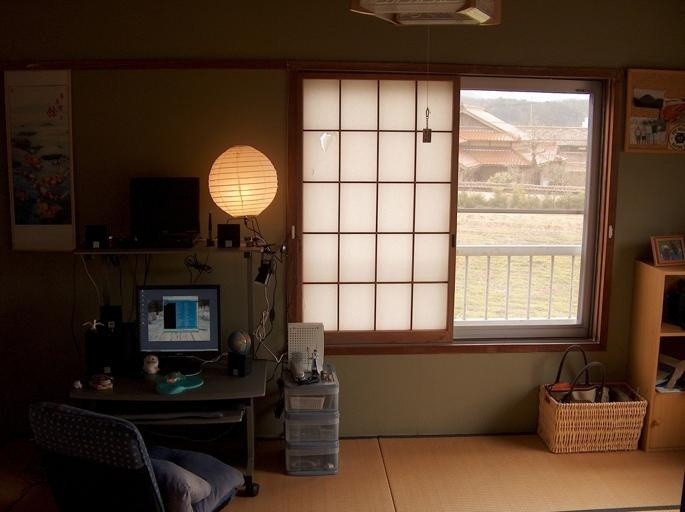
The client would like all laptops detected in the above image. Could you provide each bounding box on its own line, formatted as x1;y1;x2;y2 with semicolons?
120;175;202;249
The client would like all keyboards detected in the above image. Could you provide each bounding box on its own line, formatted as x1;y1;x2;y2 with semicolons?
111;408;224;420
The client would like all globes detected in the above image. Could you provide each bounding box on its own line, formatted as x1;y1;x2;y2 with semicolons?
227;328;251;354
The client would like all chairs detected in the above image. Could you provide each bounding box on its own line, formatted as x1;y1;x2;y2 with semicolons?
23;400;246;512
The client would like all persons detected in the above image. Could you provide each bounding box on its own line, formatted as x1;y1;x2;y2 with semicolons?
634;120;657;144
670;240;682;259
661;245;672;260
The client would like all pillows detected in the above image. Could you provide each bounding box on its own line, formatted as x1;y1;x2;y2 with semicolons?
150;459;212;511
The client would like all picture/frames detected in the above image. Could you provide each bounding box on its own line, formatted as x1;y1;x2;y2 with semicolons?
650;232;685;266
624;68;685;154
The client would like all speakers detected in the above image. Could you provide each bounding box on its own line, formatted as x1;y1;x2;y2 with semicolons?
217;223;241;249
84;223;109;250
227;352;246;378
100;304;122;330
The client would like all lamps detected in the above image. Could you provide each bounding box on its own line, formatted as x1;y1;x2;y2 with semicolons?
349;0;502;143
207;143;279;244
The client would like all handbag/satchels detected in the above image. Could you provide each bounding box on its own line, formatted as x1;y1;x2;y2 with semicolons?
547;344;610;403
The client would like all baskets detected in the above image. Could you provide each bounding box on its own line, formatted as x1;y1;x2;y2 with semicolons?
537;384;648;454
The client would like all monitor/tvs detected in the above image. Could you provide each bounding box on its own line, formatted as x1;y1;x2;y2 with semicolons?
135;283;220;377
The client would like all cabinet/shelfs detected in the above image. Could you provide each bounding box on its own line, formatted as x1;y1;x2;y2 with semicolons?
285;363;341;477
631;258;685;453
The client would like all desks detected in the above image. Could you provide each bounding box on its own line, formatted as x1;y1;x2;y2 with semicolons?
67;358;269;495
